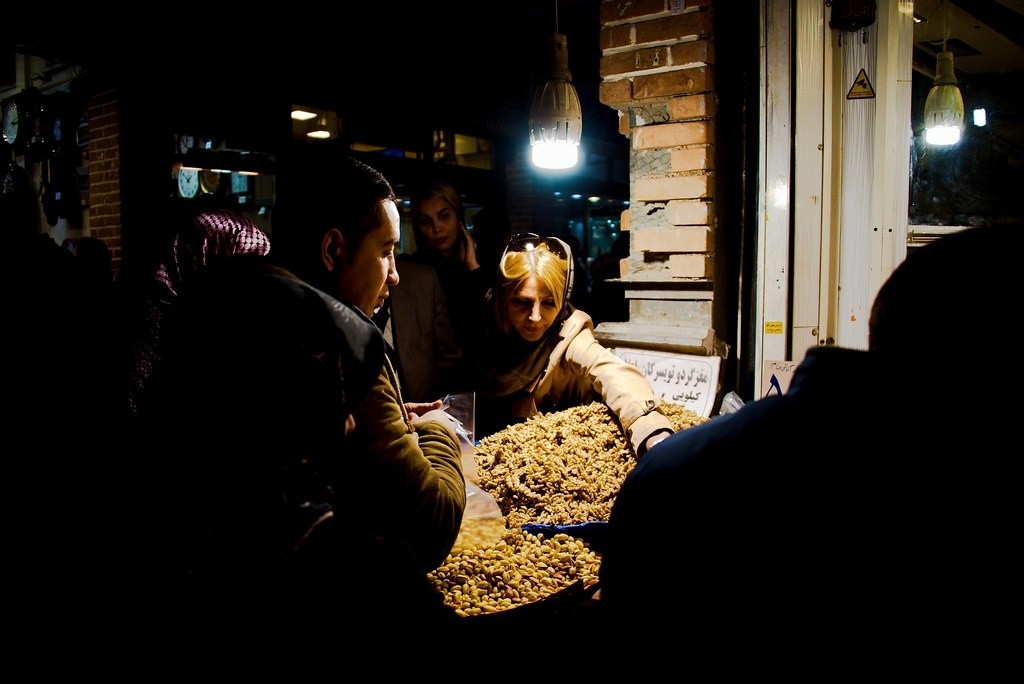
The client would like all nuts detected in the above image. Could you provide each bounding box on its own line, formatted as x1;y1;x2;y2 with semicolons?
426;519;602;617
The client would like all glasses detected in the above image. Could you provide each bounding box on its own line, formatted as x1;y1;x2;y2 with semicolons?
505;233;568;260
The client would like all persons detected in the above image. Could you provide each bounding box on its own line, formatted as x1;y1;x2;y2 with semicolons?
601;91;1024;634
127;207;271;421
408;180;499;359
464;231;677;459
371;256;463;404
130;150;469;626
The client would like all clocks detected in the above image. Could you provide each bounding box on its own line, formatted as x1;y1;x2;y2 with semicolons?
1;94;32;151
177;166;199;198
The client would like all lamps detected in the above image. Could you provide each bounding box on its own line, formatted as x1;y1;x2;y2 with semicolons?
920;0;965;154
523;0;586;180
